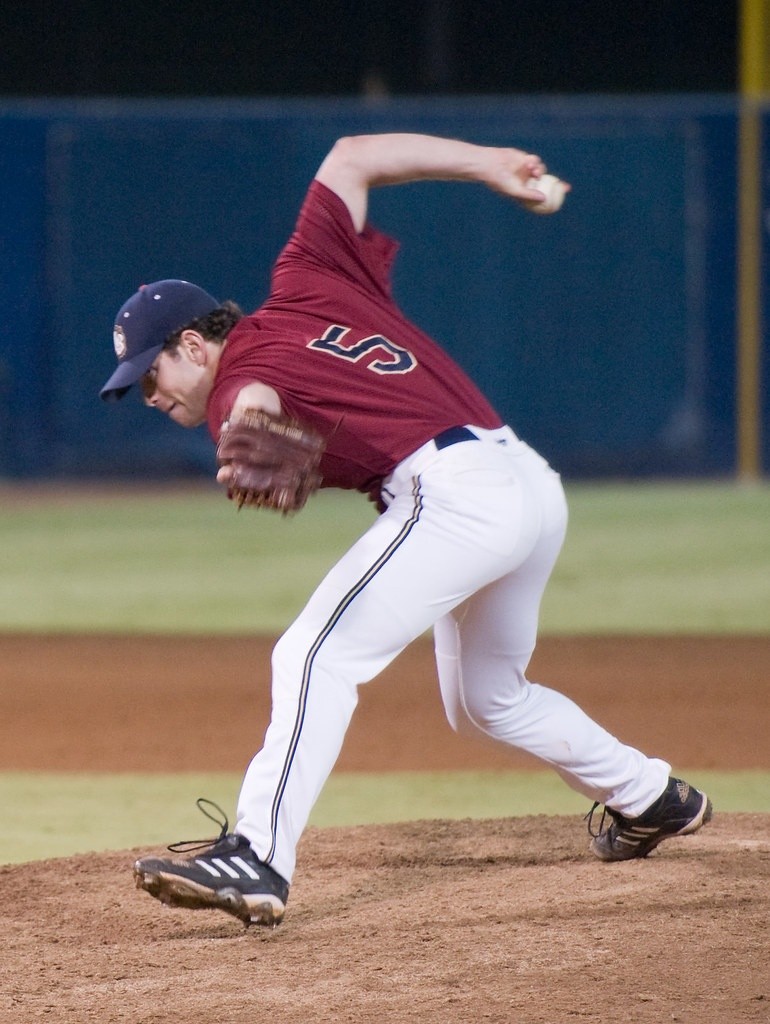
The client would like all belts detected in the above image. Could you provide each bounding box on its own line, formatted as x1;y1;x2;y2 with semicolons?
435;426;480;450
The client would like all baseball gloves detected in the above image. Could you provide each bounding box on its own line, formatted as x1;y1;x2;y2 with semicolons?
215;410;328;518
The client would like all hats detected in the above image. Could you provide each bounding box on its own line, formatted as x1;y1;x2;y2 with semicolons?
98;278;223;402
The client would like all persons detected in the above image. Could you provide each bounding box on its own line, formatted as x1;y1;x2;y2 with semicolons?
99;134;712;926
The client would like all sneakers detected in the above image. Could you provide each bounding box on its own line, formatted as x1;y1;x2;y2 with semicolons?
132;797;288;926
582;775;713;862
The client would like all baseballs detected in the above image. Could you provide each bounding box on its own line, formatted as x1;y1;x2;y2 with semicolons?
523;177;570;217
